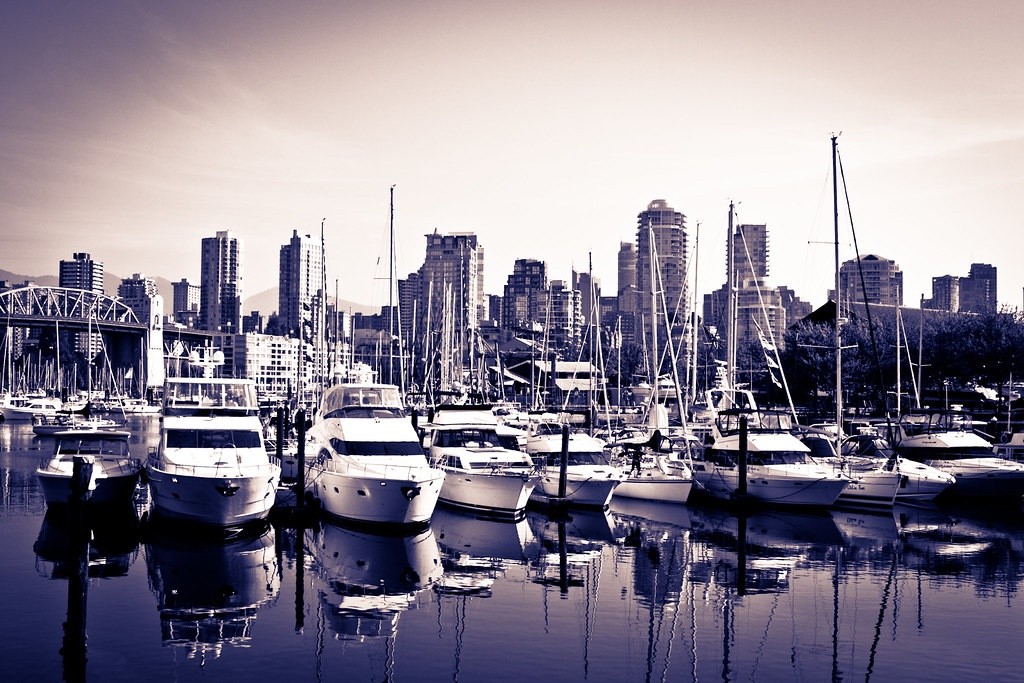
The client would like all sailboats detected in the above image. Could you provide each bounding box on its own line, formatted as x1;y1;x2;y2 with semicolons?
22;502;1024;683
4;134;1024;527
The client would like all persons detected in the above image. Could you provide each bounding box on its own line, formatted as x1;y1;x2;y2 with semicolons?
630;445;647;475
